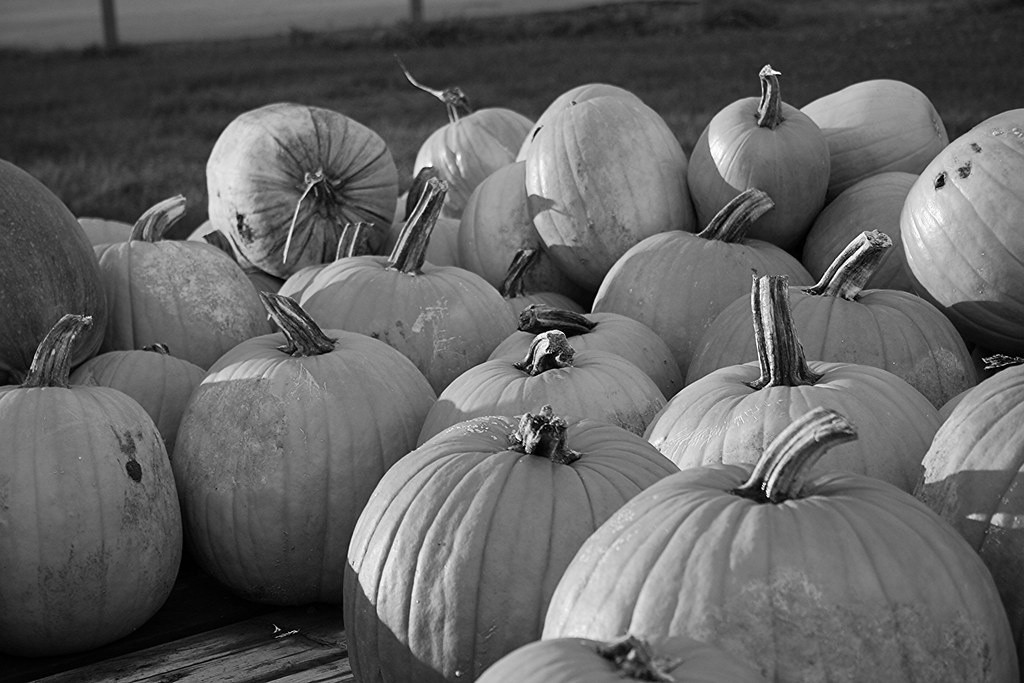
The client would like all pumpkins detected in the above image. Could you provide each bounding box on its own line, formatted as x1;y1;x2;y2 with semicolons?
0;65;1024;683
